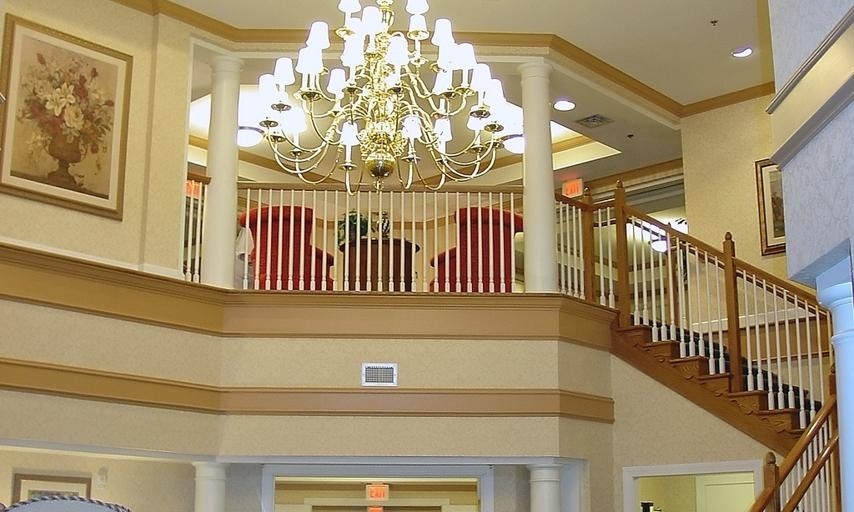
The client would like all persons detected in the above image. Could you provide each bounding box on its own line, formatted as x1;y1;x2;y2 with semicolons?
235;206;255;290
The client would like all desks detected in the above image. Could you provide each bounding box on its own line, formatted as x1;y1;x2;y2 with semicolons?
339;238;420;291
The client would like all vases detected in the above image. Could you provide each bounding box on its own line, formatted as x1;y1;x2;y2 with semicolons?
48;131;79;184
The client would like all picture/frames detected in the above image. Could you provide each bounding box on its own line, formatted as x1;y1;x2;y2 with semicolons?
0;12;133;222
755;158;786;257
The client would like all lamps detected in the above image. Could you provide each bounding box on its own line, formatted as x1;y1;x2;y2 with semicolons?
650;239;668;252
237;126;263;147
259;0;505;195
503;133;526;153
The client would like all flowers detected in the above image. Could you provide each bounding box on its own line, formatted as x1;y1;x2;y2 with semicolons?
19;51;115;170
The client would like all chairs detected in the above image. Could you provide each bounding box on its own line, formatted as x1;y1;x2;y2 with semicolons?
429;207;523;293
239;205;334;290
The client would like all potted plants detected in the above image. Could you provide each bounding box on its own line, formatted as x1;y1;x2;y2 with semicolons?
337;209;374;245
